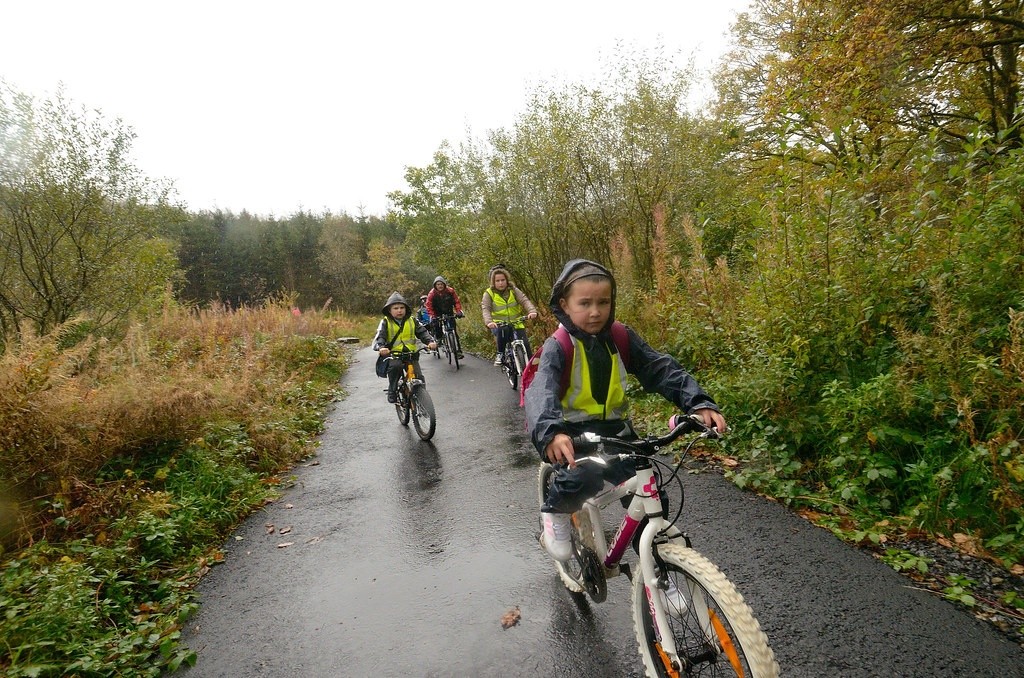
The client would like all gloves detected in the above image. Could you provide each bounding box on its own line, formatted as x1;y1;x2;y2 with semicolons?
431;318;437;326
456;311;463;319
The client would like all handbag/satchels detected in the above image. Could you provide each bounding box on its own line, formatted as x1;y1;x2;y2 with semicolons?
375;353;390;378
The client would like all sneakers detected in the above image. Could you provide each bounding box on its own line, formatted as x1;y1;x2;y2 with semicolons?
654;573;688;618
494;354;502;366
541;511;573;563
457;351;463;361
438;338;444;345
388;390;397;403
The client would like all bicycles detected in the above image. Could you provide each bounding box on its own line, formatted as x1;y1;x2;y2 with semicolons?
382;345;440;441
532;415;782;678
424;315;465;371
497;315;529;390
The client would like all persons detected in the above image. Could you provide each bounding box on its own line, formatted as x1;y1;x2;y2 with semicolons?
524;259;727;619
425;276;464;359
481;265;538;367
417;295;431;354
371;290;437;419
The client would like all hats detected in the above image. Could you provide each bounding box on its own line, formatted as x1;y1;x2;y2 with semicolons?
562;263;608;290
